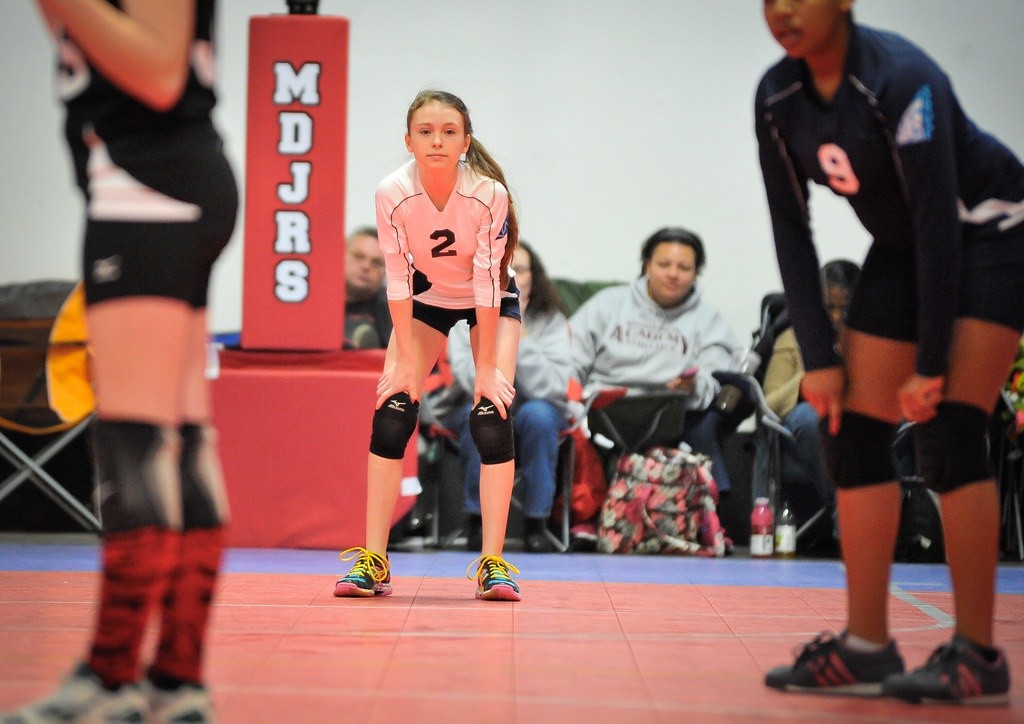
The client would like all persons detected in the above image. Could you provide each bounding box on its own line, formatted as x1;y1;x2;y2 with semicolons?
331;226;399;354
331;90;525;602
563;226;735;522
763;258;863;546
5;0;242;724
755;2;1024;704
443;238;571;554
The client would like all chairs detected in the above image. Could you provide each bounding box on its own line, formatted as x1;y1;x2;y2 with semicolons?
0;316;106;535
409;280;1024;564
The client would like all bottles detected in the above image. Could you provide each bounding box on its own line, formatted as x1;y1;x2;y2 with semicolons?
751;498;773;557
775;500;797;558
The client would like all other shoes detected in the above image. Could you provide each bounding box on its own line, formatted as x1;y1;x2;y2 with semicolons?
524;532;553;552
146;677;212;724
467;523;482;550
1;664;150;724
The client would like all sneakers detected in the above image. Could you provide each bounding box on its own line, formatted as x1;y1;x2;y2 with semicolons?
883;633;1010;704
333;546;393;596
763;629;905;697
466;554;522;601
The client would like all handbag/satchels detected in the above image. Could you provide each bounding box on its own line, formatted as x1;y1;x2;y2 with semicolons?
594;446;734;557
